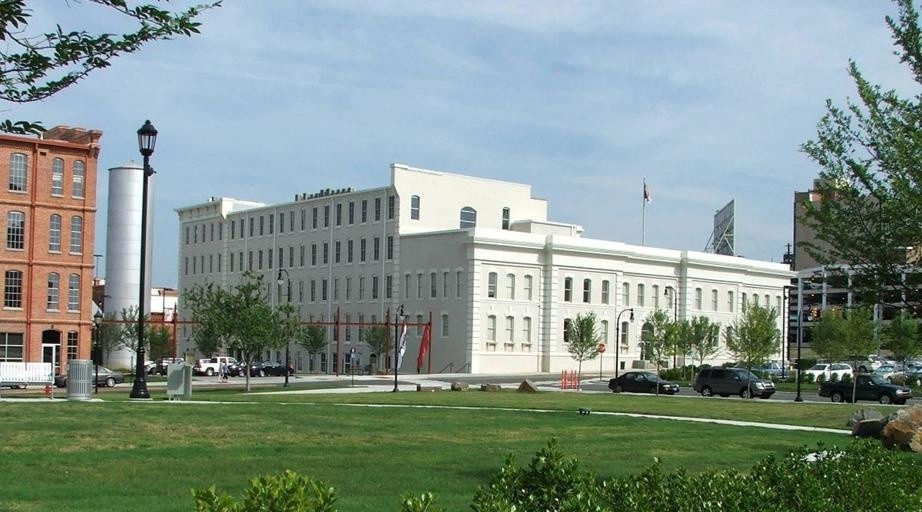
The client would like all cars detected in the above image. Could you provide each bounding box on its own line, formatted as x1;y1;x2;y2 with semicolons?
800;353;921;387
55;364;125;388
243;362;295;377
732;360;799;382
608;371;680;395
132;357;260;377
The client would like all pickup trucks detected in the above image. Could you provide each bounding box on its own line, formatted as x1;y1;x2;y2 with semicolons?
818;372;912;405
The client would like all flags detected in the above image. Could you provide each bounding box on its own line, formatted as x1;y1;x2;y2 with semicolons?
645;184;650;202
417;326;430;371
396;325;407;370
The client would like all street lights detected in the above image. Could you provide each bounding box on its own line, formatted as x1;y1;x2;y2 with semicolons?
93;310;104;393
94;253;103;279
613;308;635;393
129;119;159;399
277;268;291;387
663;286;677;368
794;300;814;401
394;304;406;392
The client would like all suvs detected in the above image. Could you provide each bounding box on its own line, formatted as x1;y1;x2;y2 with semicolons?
692;366;775;399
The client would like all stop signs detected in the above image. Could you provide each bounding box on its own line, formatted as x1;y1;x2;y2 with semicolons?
598;344;606;353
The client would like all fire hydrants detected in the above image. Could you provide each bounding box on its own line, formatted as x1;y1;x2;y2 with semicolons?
44;384;53;396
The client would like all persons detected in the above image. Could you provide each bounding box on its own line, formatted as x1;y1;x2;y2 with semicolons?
222;364;230;381
218;361;224;383
